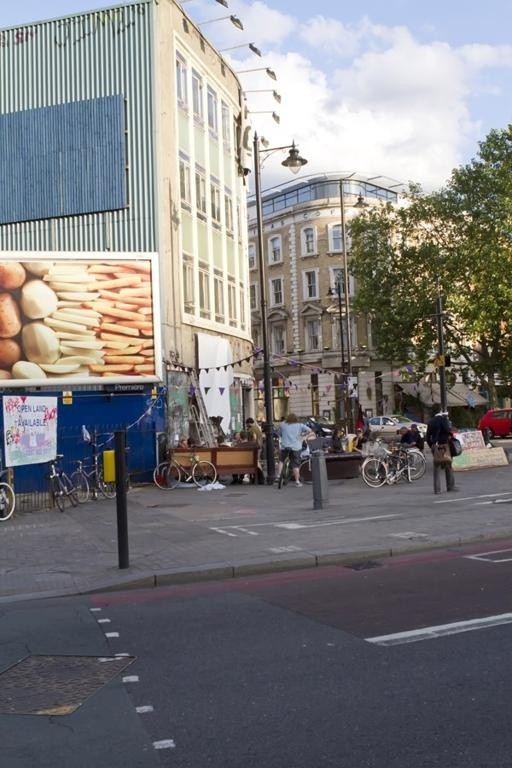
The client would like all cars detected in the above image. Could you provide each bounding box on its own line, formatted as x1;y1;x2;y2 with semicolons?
370;415;429;441
479;409;512;438
298;416;336;439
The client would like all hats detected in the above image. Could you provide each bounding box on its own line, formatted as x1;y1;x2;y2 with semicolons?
356;428;363;433
409;424;417;428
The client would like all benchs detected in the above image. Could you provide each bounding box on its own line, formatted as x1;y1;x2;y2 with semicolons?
304;437;369;479
165;446;261;485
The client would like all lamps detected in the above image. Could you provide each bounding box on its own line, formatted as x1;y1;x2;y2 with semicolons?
245;89;281;103
220;41;262;57
177;0;229;8
245;110;280;124
199;13;244;30
235;66;277;81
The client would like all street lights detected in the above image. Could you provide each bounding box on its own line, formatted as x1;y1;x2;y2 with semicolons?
339;180;369;435
253;130;308;484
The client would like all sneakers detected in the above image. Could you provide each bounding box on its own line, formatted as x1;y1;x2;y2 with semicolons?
296;480;302;488
447;486;461;492
275;475;284;482
231;479;243;484
435;489;442;495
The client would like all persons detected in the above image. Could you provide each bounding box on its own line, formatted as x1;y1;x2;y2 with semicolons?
410;423;421;435
391;425;424;452
424;401;461;494
176;412;372;488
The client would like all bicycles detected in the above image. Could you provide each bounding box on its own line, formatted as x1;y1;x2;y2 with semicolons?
49;455;78;512
278;447;293;489
0;469;16;521
71;453;117;504
153;444;216;490
361;441;427;487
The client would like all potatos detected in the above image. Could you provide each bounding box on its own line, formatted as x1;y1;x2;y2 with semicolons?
0;260;154;380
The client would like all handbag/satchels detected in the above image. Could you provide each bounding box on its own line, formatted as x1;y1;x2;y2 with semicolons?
433;445;453;462
450;438;463;456
